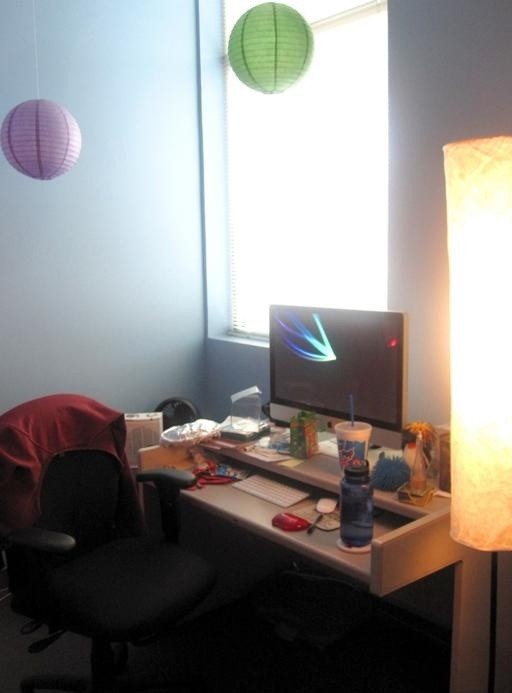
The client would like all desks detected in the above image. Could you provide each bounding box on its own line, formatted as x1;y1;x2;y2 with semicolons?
137;423;488;690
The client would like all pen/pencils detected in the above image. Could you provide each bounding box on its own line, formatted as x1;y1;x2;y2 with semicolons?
308;513;323;535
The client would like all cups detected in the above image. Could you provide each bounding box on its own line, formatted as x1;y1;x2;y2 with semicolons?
335;420;374;471
439;432;452;493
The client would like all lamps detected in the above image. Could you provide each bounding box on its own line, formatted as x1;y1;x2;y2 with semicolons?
439;134;511;692
225;0;315;92
0;1;84;183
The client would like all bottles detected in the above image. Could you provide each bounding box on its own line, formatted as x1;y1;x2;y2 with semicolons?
409;431;426;498
339;456;376;545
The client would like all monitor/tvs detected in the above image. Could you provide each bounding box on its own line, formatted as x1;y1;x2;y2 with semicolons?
268;303;409;460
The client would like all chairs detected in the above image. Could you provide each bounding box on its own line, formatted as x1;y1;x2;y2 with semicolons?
2;393;221;692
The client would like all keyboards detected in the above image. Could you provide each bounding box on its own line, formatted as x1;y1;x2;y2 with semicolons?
232;474;312;509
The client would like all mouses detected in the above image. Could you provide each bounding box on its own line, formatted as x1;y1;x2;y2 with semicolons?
316;495;338;514
